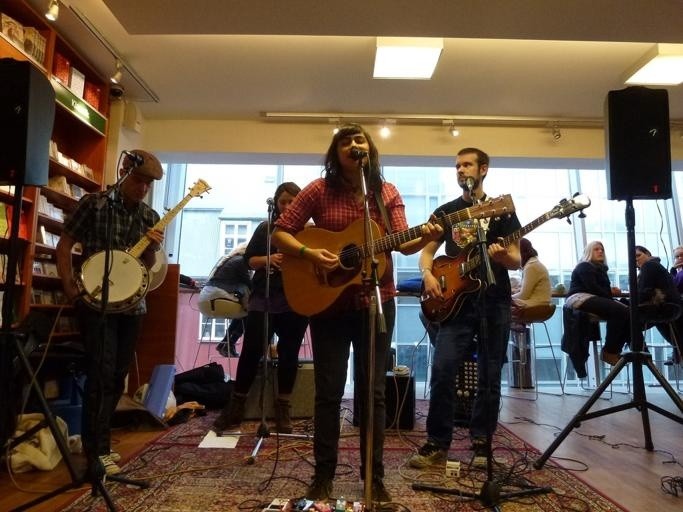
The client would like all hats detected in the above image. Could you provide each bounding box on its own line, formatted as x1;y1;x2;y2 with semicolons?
123;150;162;180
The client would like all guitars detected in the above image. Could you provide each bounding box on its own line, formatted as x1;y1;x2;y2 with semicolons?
419;193;591;327
282;194;517;316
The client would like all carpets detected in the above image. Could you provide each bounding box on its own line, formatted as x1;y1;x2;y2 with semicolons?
53;398;633;511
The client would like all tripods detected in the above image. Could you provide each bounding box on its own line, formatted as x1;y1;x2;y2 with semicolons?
215;209;309;465
533;198;683;471
7;197;150;512
0;186;82;489
412;241;553;512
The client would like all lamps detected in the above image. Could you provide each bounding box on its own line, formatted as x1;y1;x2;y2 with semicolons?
264;112;561;140
369;37;443;81
621;43;683;86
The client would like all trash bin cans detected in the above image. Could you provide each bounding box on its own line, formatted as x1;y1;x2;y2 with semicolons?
509;326;533;389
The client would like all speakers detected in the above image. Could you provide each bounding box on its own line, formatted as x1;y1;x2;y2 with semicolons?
603;87;672;199
0;57;55;186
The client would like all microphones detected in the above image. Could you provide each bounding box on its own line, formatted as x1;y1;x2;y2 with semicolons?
124;150;146;167
466;175;476;201
267;198;275;206
349;148;368;162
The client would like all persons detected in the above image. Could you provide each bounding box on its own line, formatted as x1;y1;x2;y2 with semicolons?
508;237;552;324
406;145;523;470
268;121;447;503
655;246;683;366
211;182;308;435
568;240;629;367
634;245;682;353
52;148;166;478
197;239;255;361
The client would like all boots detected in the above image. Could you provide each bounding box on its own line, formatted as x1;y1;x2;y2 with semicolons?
277;398;294;435
214;393;248;427
216;332;239;356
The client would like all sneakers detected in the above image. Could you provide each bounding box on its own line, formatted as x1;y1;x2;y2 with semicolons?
409;443;447;468
364;476;393;506
474;439;487;469
307;475;333;500
98;451;122;475
599;347;623;367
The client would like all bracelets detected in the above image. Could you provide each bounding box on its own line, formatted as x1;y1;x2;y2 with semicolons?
299;244;307;258
420;267;432;280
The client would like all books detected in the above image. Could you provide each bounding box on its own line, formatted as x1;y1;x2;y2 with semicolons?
53;51;100;112
38;195;64;221
0;202;77;332
49;140;95;181
0;185;15;194
2;13;46;65
48;177;90;199
